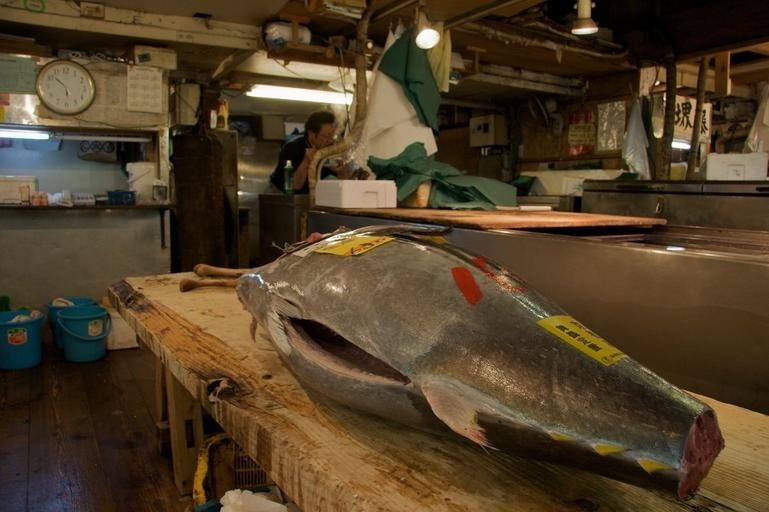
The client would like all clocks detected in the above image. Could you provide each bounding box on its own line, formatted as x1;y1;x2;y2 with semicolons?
35;59;96;115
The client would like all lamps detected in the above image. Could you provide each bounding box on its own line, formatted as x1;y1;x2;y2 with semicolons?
0;129;54;140
411;9;440;50
54;135;152;142
567;1;599;35
241;81;354;106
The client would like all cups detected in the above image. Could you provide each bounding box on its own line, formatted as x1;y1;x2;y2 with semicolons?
20;185;30;206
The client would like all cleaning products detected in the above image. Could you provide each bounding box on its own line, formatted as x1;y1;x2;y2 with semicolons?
283;159;293;197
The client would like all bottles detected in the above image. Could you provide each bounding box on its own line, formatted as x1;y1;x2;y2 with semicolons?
32;191;48;207
283;160;295;201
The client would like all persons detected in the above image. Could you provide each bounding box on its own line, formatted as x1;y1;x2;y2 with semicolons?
248;107;353;271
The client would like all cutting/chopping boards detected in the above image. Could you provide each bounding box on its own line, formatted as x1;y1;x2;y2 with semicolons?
312;209;668;233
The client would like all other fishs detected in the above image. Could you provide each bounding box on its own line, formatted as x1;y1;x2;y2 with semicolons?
236;225;725;501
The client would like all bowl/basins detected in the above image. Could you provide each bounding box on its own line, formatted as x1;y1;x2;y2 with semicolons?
107;191;136;206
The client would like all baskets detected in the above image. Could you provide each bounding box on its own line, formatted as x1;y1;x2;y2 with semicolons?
206;440;275;496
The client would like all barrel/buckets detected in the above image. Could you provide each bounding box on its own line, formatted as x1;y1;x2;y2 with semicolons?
57;306;113;363
126;162;160;206
46;295;98;345
0;306;47;371
